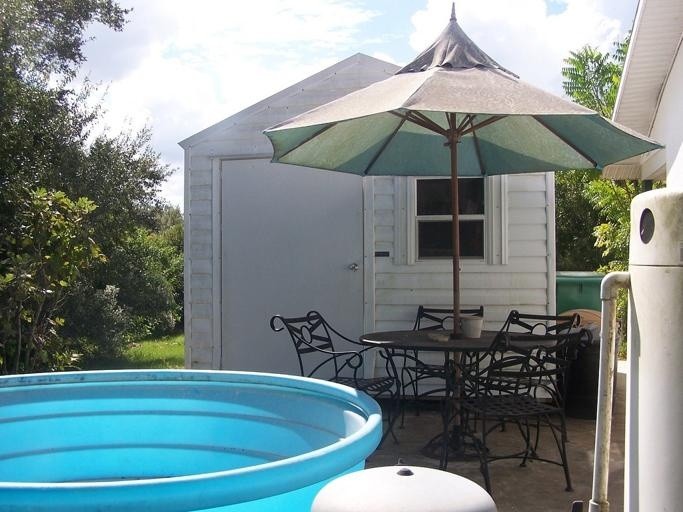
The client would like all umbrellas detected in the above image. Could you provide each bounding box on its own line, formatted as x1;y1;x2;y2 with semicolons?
260;3;666;450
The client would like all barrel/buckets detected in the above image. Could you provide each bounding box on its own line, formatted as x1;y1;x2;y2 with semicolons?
462;315;482;339
0;368;382;512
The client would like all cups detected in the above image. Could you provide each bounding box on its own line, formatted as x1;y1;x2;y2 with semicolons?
462;317;483;339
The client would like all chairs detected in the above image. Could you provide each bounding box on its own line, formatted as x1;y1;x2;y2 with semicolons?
269;310;399;463
440;327;593;497
470;309;580;457
397;303;485;431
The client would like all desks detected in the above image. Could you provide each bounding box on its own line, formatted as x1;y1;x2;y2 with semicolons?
359;326;566;458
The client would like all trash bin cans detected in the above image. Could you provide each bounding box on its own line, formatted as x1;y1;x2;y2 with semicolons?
556;310;617;419
